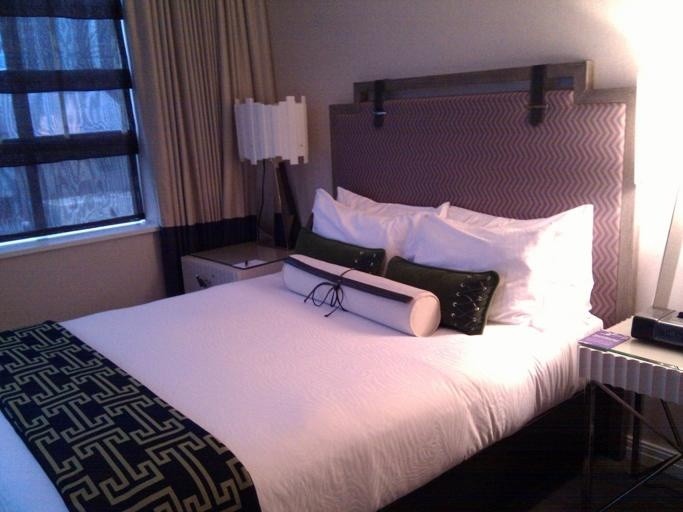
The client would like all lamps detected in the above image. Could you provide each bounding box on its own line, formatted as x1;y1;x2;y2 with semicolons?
233;95;309;248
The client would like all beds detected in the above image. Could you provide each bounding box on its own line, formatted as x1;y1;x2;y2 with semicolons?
2;62;634;507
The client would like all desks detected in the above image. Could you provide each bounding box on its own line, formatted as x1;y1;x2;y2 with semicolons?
581;314;683;511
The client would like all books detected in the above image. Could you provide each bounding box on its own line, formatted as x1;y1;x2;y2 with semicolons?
579;329;630;353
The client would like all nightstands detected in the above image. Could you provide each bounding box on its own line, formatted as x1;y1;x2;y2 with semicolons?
181;242;292;293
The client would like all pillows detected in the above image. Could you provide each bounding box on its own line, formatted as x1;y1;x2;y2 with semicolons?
284;184;594;340
